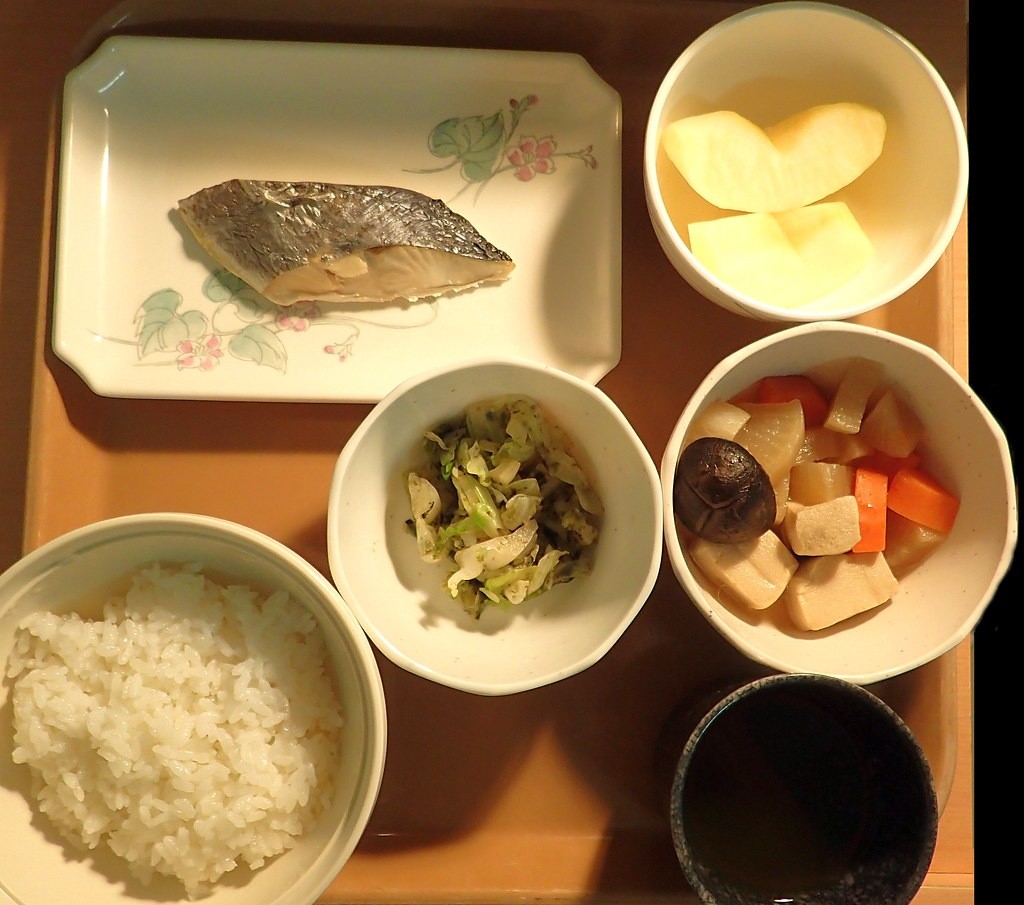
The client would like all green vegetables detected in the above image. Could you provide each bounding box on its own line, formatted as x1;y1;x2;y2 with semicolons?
404;394;601;620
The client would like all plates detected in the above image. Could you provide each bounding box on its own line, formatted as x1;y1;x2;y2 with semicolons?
53;35;622;404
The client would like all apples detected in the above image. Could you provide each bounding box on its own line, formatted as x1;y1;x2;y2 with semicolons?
663;100;887;214
687;200;876;309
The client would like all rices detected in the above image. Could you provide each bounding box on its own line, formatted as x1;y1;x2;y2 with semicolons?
2;561;344;901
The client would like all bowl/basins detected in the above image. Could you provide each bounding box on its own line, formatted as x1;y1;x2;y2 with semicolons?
660;319;1019;684
0;514;388;905
643;1;969;322
668;674;938;905
327;359;664;697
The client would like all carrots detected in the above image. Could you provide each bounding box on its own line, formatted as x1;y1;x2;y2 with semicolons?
754;375;962;553
